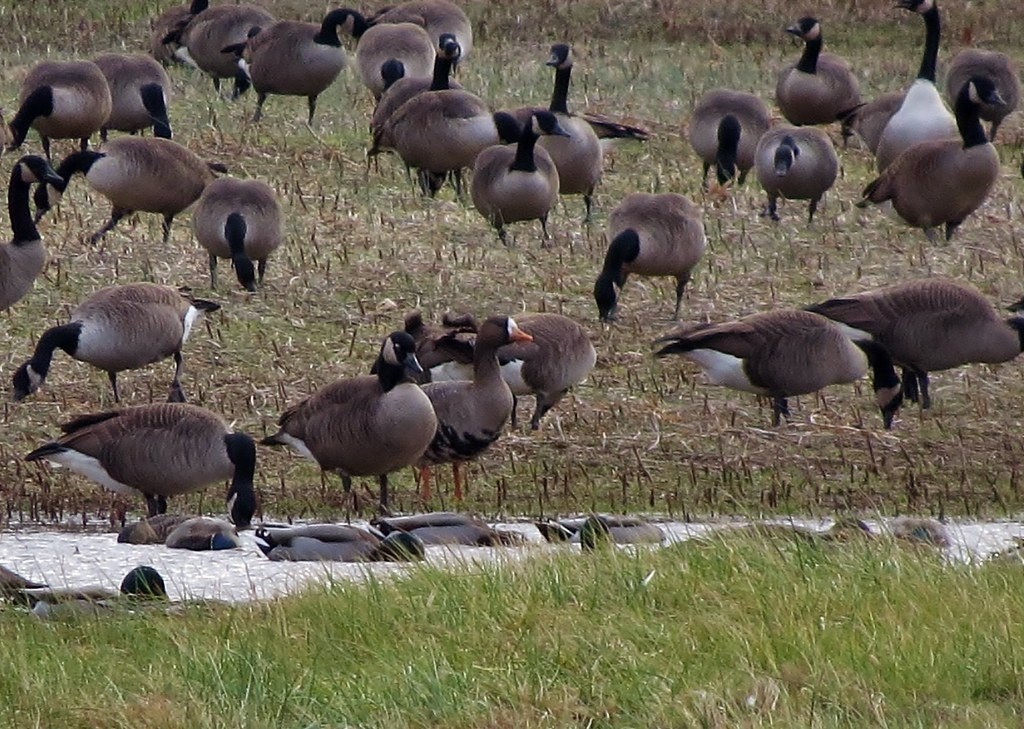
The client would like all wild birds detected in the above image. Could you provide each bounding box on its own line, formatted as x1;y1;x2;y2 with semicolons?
593;192;707;323
648;277;1024;432
152;1;649;251
12;281;256;532
687;0;1024;243
256;308;598;516
0;51;285;314
